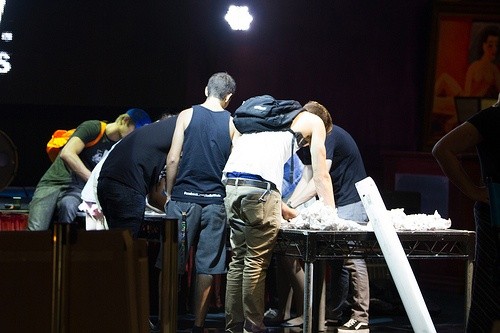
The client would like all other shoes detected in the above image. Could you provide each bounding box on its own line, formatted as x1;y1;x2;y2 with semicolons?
280;316;303;327
264;308;279;319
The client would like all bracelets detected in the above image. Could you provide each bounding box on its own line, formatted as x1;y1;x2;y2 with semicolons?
287;204;292;210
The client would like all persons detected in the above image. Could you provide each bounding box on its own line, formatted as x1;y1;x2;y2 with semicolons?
26;109;151;232
431;93;500;333
81;73;370;333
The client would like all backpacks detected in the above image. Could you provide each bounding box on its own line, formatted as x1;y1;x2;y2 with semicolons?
45;121;106;163
233;95;308;148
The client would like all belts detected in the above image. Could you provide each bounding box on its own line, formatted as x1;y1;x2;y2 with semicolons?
226;179;276;190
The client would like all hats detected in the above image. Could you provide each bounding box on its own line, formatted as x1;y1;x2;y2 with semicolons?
126;108;153;129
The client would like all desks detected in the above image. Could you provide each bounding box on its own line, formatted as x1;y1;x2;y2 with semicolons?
267;230;478;333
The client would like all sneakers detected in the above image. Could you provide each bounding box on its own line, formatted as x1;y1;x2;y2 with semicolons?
338;319;369;333
